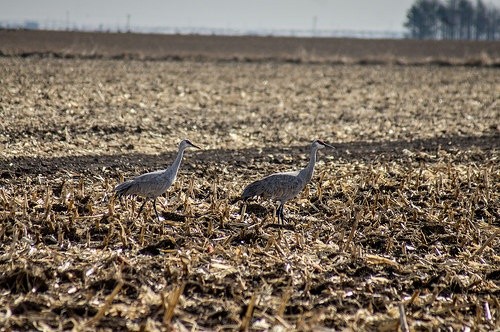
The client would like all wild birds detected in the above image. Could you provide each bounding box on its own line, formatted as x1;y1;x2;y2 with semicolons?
114;139;201;221
241;140;335;224
